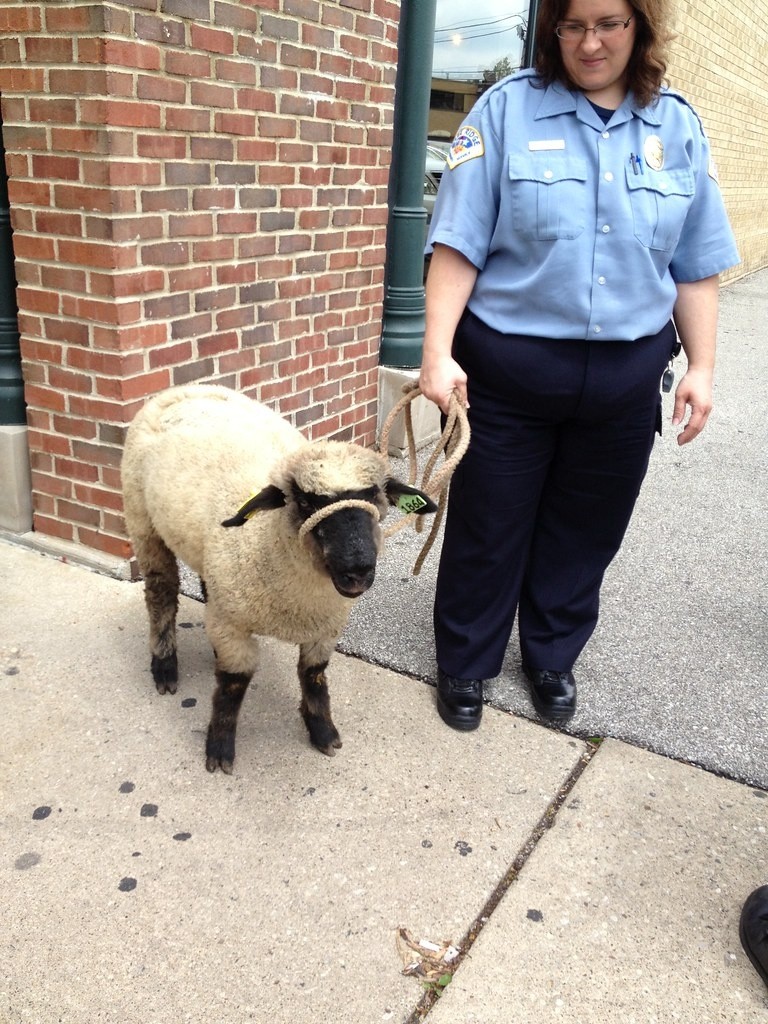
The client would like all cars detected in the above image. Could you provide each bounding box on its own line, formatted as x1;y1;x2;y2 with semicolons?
424;141;452;281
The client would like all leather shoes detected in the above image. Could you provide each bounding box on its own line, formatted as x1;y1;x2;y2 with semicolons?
521;654;576;718
435;664;483;729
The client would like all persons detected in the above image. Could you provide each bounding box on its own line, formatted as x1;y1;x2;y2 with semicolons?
416;0;742;734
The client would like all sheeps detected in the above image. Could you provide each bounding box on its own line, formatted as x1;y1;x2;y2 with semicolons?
119;383;440;774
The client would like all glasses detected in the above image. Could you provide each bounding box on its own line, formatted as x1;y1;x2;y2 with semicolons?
554;12;636;40
